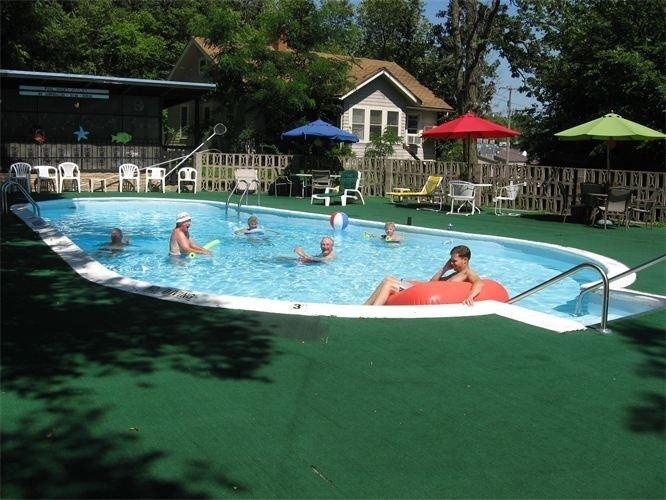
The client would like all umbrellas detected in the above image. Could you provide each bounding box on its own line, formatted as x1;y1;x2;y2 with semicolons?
281;121;359;198
418;112;521;216
555;112;666;227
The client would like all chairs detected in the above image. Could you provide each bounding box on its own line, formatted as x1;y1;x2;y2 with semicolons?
7;161;201;197
557;179;663;231
270;165;528;220
234;167;259;196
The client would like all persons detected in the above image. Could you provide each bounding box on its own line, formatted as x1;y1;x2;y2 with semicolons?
362;245;484;307
169;211;209;257
293;237;337;264
380;221;400;243
236;216;259;236
102;228;129;246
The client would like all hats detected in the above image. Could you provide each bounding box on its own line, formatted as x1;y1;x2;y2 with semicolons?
175;212;194;224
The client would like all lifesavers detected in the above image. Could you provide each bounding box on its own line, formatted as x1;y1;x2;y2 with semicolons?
385;279;509;305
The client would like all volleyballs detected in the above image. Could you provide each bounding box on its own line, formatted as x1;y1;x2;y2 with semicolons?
330;212;350;229
